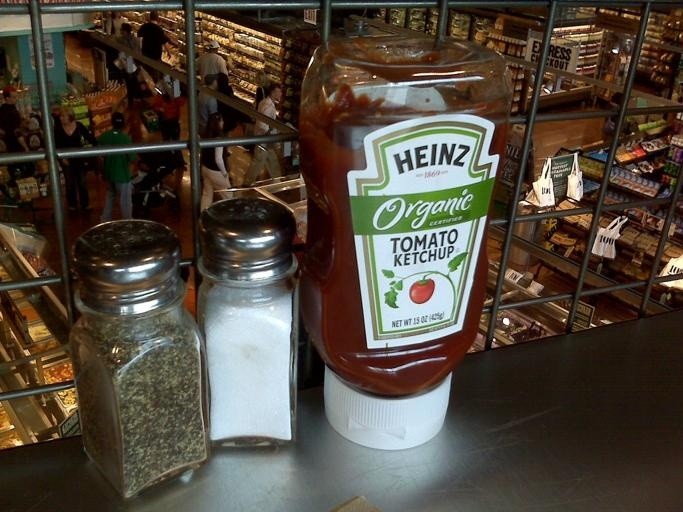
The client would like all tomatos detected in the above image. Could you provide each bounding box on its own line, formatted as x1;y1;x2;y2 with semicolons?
409;279;435;304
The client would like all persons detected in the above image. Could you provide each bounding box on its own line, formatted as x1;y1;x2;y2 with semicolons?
179;264;198;319
0;11;278;225
592;93;632;136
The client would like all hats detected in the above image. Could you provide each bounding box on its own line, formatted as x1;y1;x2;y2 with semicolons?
209;40;221;49
204;74;218;84
111;112;125;130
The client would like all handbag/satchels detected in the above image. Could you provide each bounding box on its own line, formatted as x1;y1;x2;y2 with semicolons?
523;157;555;207
566;153;583;201
657;255;682;290
590;216;628;258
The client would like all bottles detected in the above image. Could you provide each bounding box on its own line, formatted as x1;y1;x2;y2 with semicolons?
194;195;300;449
69;218;208;501
298;31;517;454
